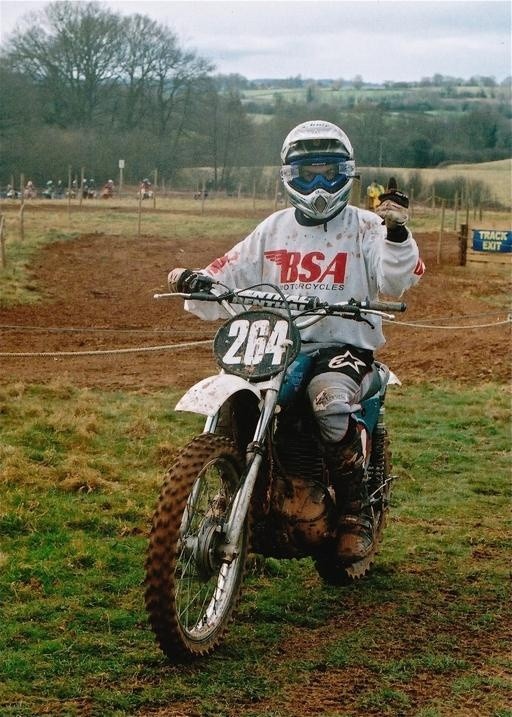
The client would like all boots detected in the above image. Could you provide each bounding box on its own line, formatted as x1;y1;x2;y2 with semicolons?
321;415;376;561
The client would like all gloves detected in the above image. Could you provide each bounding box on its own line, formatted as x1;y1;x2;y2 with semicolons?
168;268;204;292
373;177;410;243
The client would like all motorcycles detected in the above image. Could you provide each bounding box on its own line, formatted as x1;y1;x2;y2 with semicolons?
135;186;154;200
5;186;113;200
140;267;409;662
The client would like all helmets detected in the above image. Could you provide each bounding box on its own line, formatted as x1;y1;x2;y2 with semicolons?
280;121;357;220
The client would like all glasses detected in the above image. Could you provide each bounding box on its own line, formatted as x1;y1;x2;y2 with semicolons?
278;157;355;187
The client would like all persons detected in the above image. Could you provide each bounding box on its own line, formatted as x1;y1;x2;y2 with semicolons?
140;179;153;192
367;178;385;209
167;120;427;564
6;178;115;198
